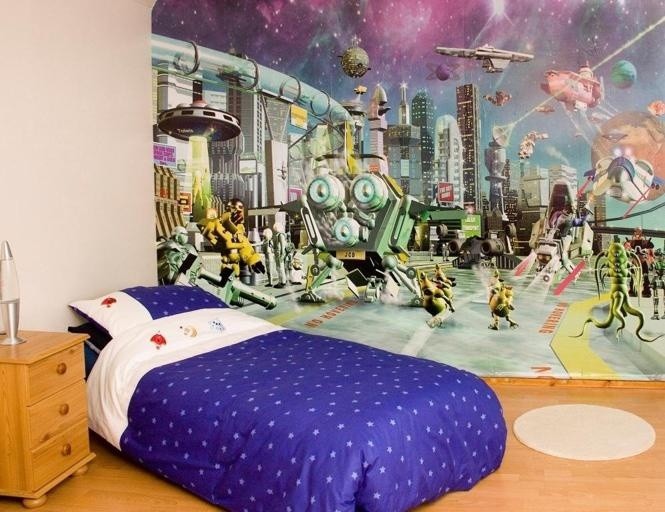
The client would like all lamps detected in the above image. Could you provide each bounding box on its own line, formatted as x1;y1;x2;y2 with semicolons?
0;240;26;345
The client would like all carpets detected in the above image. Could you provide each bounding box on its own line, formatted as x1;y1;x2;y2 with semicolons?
512;403;656;460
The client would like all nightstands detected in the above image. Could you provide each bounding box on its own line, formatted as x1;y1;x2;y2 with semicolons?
0;328;96;508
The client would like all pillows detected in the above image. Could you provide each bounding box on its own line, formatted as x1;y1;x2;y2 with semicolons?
68;285;229;339
68;318;111;352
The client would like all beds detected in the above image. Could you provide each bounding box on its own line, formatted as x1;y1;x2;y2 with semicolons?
83;308;506;512
